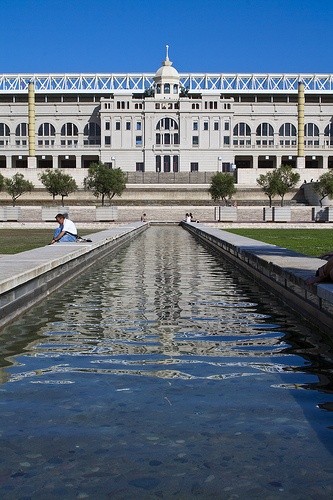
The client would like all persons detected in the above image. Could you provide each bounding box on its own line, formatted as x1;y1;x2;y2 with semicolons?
141;213;147;222
49;213;77;245
183;212;194;222
304;252;333;286
